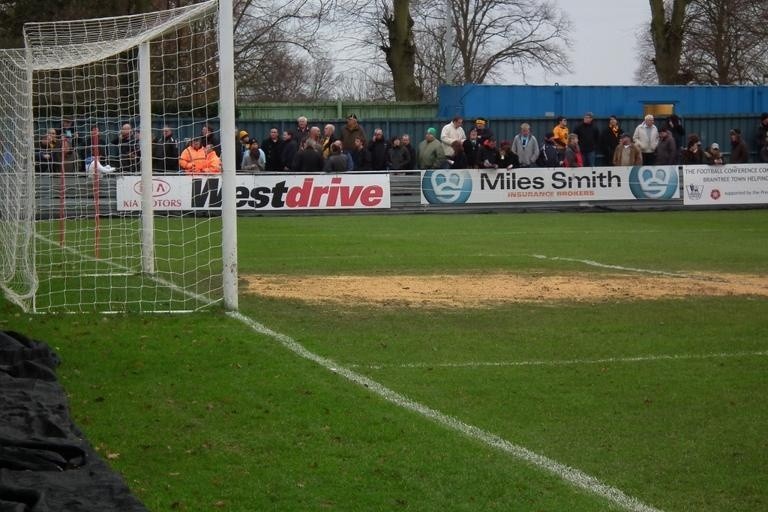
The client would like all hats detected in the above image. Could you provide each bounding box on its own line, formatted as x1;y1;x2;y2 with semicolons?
712;143;719;150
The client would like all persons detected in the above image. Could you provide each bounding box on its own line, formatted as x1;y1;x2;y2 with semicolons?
36;122;224;174
234;114;752;175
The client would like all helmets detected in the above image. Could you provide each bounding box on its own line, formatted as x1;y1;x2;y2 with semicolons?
240;131;249;139
